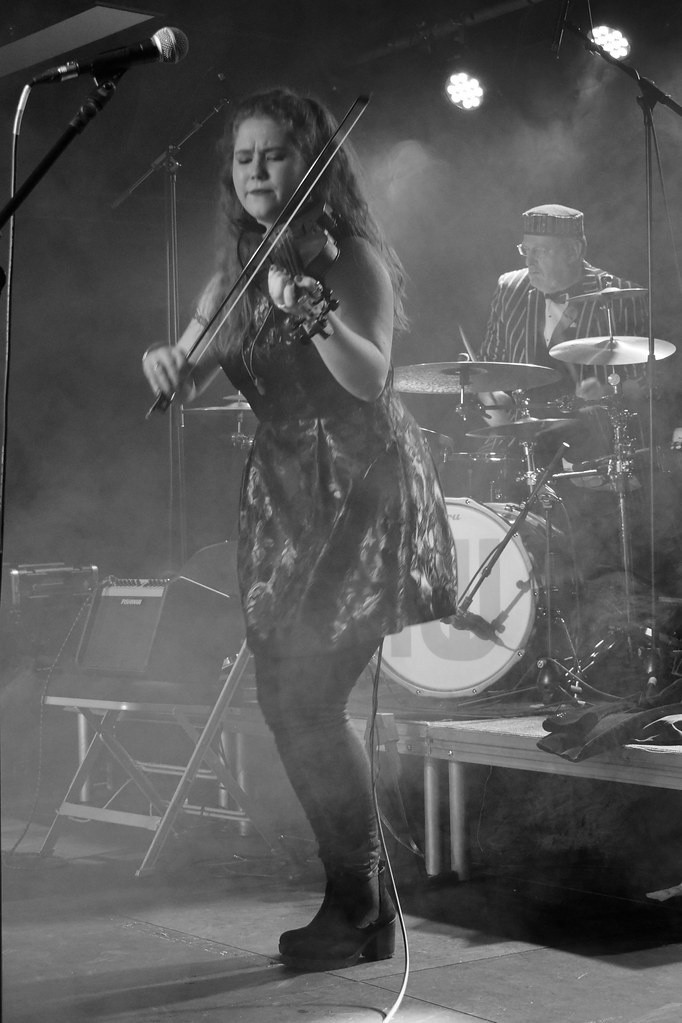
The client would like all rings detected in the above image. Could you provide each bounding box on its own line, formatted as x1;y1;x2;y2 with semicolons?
153;362;164;371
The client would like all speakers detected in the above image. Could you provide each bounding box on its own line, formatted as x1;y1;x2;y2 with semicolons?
74;577;230;684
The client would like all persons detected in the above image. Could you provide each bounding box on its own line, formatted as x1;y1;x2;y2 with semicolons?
477;204;660;592
143;88;458;972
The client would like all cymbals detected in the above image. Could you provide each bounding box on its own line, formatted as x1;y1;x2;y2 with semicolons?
220;394;249;402
548;334;677;367
445;449;513;465
417;425;455;454
565;286;650;303
464;416;584;440
178;401;256;415
391;360;562;396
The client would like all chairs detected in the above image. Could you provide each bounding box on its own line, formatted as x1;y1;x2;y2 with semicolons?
39;540;298;879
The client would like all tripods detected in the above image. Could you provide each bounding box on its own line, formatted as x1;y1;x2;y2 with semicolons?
456;19;682;710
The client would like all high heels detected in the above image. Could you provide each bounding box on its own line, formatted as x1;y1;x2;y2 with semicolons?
278;866;399;972
276;857;386;954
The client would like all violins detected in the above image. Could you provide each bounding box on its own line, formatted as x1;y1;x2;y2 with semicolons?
236;194;344;344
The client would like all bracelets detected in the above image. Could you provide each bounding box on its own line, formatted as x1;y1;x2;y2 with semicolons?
142;342;176;364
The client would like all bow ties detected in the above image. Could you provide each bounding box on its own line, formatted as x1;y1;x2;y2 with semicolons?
544;293;566;305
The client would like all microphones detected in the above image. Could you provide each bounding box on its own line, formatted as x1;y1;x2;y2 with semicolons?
443;613;480;631
552;0;570;60
32;26;188;83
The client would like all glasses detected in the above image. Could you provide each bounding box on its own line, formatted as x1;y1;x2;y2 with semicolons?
517;240;578;259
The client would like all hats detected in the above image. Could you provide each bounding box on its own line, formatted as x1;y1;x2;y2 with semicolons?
521;203;585;237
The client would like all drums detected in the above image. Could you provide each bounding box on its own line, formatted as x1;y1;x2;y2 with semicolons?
368;495;545;700
480;502;572;696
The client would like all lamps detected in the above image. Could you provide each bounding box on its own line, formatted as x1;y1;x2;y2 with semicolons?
585;0;645;63
441;32;515;112
